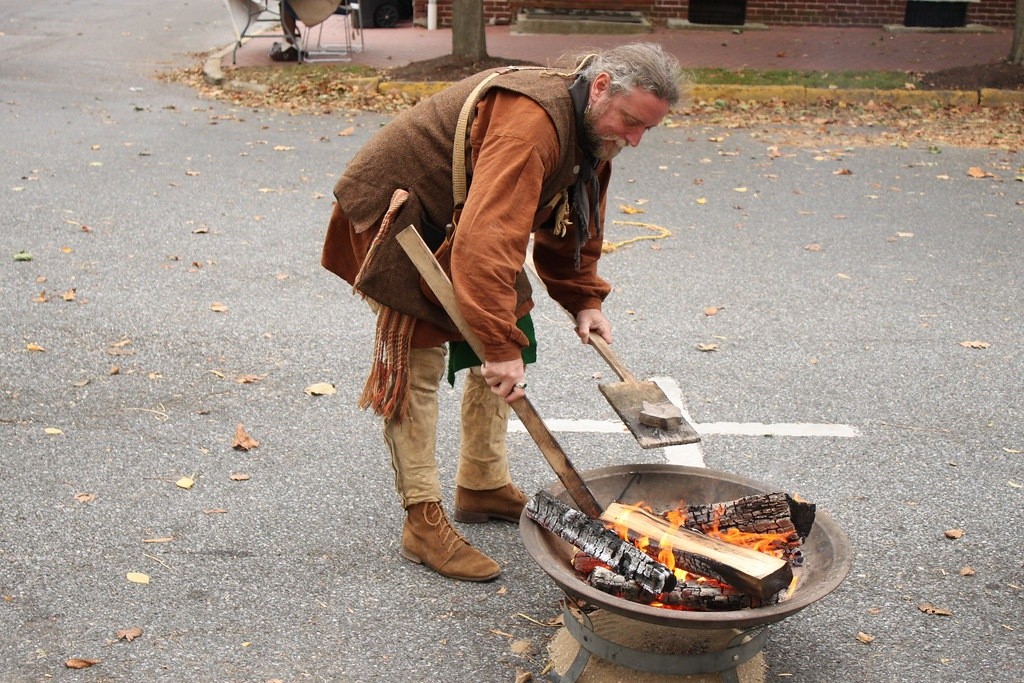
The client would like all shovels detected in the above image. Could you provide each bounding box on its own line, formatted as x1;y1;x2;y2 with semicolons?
525;250;701;449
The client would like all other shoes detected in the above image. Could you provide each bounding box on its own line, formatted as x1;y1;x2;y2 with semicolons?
455;480;532;524
271;46;309;61
400;502;501;582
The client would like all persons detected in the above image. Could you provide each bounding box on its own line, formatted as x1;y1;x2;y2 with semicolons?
323;43;679;583
270;0;342;61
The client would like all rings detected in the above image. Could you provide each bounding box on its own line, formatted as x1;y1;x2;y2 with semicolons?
516;383;527;387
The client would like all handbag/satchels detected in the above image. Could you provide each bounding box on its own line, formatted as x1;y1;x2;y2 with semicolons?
420;215;470;304
